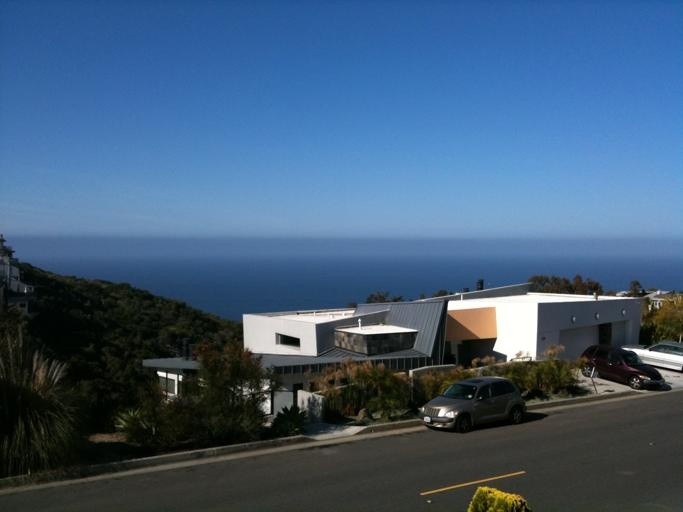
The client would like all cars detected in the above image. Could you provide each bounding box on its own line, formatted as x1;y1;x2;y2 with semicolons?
577;344;665;390
620;339;683;372
419;376;528;433
